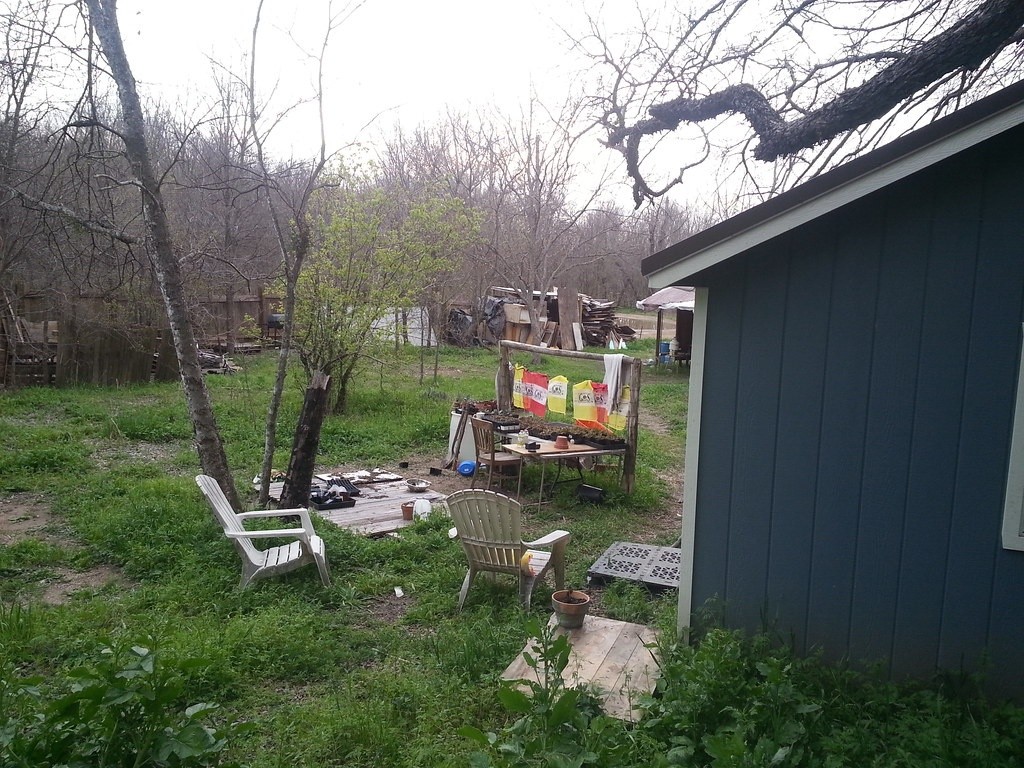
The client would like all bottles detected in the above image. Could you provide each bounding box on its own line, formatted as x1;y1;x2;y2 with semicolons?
473;399;497;411
485;409;520;435
517;429;529;448
453;397;467;408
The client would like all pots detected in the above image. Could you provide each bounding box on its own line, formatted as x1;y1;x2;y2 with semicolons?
403;478;432;492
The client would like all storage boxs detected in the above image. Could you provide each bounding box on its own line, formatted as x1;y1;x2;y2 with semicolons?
576;420;613;433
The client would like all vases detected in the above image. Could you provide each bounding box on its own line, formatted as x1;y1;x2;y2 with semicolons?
554;436;568;449
401;505;414;520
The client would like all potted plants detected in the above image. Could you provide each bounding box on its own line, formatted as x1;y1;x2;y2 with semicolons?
551;584;590;628
484;413;625;447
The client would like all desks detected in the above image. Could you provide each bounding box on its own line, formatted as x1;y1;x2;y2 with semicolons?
493;431;584;497
501;443;626;515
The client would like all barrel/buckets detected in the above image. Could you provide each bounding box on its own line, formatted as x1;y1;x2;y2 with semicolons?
660;343;670;363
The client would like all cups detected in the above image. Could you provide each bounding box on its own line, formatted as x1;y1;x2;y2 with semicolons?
473;412;485;419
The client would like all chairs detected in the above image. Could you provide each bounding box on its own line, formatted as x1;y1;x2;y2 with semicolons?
446;489;572;613
469;417;522;492
195;475;332;590
